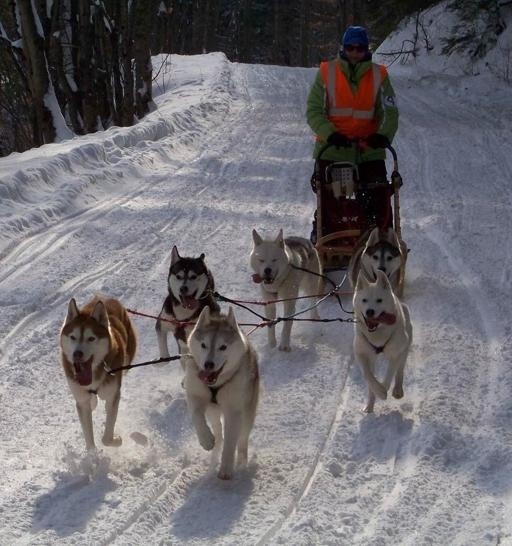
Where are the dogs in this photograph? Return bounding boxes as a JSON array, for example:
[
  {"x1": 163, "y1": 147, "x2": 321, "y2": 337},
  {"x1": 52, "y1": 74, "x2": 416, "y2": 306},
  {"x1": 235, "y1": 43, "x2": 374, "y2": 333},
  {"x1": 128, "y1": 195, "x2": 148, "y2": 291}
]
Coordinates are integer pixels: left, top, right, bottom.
[
  {"x1": 153, "y1": 245, "x2": 220, "y2": 371},
  {"x1": 181, "y1": 305, "x2": 261, "y2": 480},
  {"x1": 347, "y1": 227, "x2": 404, "y2": 299},
  {"x1": 249, "y1": 228, "x2": 321, "y2": 352},
  {"x1": 352, "y1": 268, "x2": 413, "y2": 413},
  {"x1": 59, "y1": 294, "x2": 137, "y2": 464}
]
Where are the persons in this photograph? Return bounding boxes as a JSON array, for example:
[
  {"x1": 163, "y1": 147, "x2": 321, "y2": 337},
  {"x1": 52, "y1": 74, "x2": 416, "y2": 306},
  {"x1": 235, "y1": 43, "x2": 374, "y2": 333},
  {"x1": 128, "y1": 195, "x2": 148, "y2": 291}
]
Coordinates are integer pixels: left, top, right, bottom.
[{"x1": 305, "y1": 25, "x2": 399, "y2": 244}]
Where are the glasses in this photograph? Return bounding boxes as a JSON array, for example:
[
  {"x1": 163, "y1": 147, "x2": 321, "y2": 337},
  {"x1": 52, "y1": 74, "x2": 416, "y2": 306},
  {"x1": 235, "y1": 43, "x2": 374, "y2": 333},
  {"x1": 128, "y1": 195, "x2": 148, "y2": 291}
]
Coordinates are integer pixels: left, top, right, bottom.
[{"x1": 344, "y1": 46, "x2": 366, "y2": 53}]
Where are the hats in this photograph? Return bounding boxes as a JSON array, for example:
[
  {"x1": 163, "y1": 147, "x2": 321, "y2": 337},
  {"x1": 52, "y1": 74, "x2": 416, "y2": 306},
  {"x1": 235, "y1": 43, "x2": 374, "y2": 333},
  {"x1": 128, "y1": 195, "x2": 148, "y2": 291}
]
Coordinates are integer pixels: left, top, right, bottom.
[{"x1": 342, "y1": 26, "x2": 369, "y2": 46}]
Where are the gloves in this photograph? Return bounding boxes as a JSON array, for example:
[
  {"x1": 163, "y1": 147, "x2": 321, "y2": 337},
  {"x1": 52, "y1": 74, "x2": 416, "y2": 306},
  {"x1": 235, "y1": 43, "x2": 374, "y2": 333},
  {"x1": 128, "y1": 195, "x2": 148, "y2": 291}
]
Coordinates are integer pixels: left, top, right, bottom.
[
  {"x1": 367, "y1": 133, "x2": 390, "y2": 148},
  {"x1": 327, "y1": 131, "x2": 352, "y2": 148}
]
